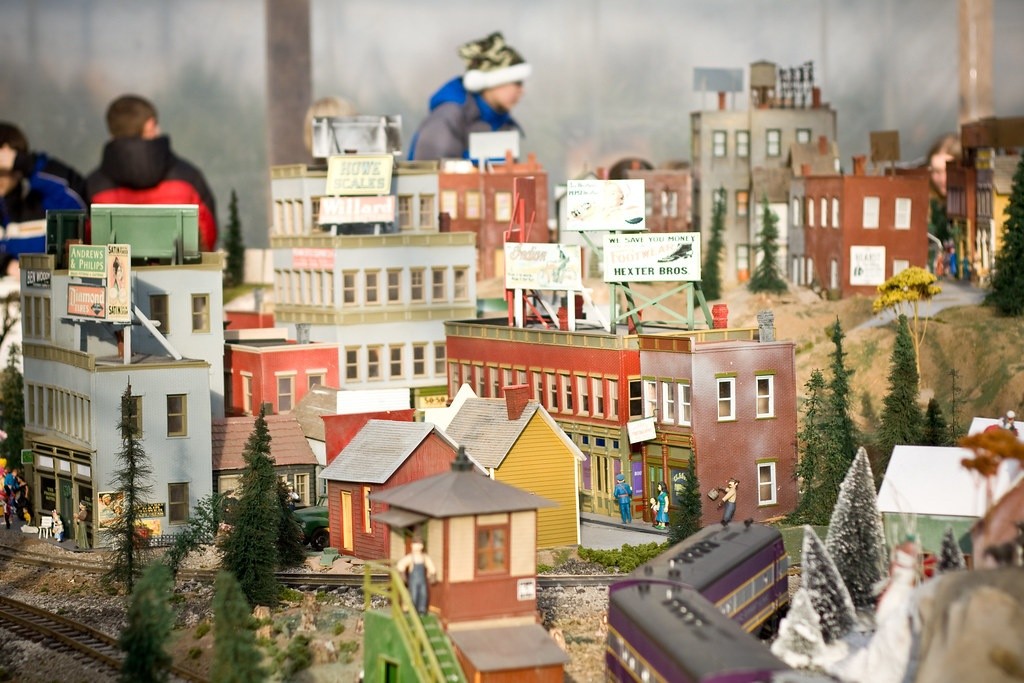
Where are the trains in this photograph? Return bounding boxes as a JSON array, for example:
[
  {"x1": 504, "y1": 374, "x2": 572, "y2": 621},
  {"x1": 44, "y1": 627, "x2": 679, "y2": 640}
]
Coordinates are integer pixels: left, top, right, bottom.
[{"x1": 604, "y1": 522, "x2": 795, "y2": 683}]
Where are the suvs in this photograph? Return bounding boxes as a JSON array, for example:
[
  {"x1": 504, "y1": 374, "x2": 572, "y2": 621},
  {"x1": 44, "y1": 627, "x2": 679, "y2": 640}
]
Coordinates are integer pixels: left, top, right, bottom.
[{"x1": 292, "y1": 494, "x2": 329, "y2": 551}]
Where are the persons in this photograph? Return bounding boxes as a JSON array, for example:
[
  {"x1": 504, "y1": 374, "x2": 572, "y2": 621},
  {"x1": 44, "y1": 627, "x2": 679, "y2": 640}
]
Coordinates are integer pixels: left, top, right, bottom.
[
  {"x1": 0, "y1": 93, "x2": 359, "y2": 284},
  {"x1": 717, "y1": 477, "x2": 740, "y2": 524},
  {"x1": 4, "y1": 469, "x2": 90, "y2": 549},
  {"x1": 927, "y1": 132, "x2": 960, "y2": 275},
  {"x1": 615, "y1": 475, "x2": 633, "y2": 524},
  {"x1": 408, "y1": 31, "x2": 530, "y2": 162},
  {"x1": 934, "y1": 246, "x2": 975, "y2": 283},
  {"x1": 395, "y1": 539, "x2": 437, "y2": 615},
  {"x1": 650, "y1": 481, "x2": 669, "y2": 530}
]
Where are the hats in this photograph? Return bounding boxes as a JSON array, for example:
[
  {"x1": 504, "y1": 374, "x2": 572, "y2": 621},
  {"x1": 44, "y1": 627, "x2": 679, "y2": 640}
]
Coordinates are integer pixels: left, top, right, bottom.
[{"x1": 456, "y1": 30, "x2": 534, "y2": 92}]
